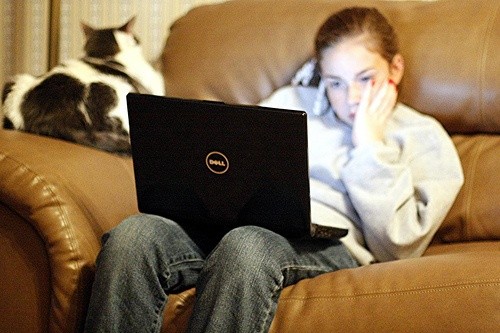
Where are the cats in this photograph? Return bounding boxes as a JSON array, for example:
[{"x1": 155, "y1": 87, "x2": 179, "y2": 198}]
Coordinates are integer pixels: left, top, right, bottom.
[{"x1": 2, "y1": 13, "x2": 166, "y2": 157}]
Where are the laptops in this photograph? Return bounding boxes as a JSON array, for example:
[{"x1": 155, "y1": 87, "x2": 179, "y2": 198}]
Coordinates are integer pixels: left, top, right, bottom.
[{"x1": 125, "y1": 92, "x2": 348, "y2": 247}]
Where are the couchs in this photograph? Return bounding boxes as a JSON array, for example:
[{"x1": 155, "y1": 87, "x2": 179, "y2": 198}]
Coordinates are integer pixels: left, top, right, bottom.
[{"x1": 0, "y1": 0, "x2": 499, "y2": 332}]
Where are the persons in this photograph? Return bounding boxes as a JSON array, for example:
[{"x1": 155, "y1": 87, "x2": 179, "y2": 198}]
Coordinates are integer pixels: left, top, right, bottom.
[{"x1": 83, "y1": 8, "x2": 464, "y2": 333}]
